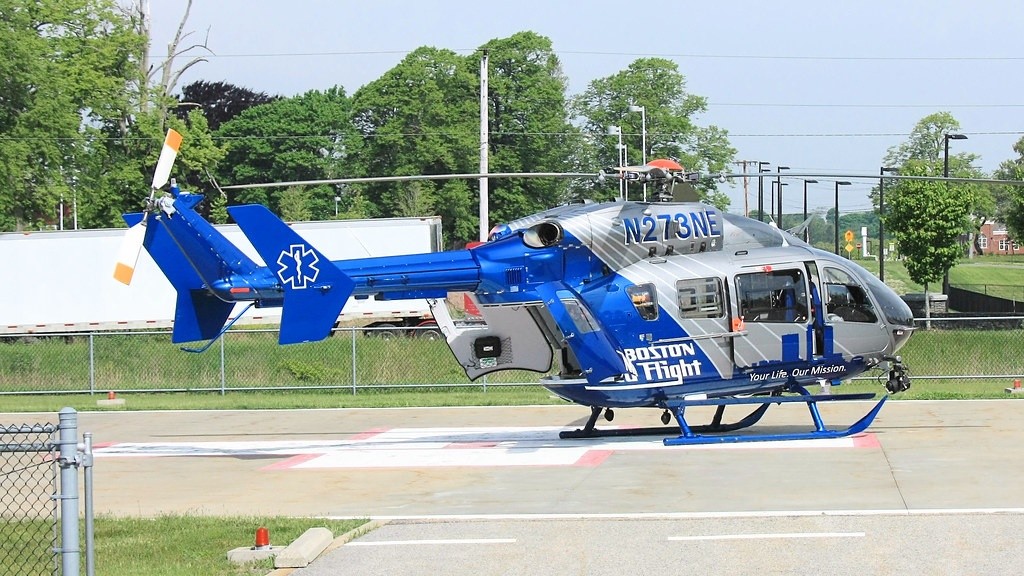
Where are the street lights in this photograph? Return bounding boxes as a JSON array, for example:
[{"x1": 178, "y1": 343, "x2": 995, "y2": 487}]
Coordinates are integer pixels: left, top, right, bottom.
[
  {"x1": 804, "y1": 180, "x2": 819, "y2": 244},
  {"x1": 879, "y1": 167, "x2": 899, "y2": 283},
  {"x1": 616, "y1": 145, "x2": 628, "y2": 203},
  {"x1": 608, "y1": 125, "x2": 629, "y2": 201},
  {"x1": 771, "y1": 166, "x2": 789, "y2": 231},
  {"x1": 835, "y1": 181, "x2": 853, "y2": 254},
  {"x1": 630, "y1": 106, "x2": 647, "y2": 201},
  {"x1": 943, "y1": 134, "x2": 969, "y2": 312},
  {"x1": 758, "y1": 162, "x2": 770, "y2": 221}
]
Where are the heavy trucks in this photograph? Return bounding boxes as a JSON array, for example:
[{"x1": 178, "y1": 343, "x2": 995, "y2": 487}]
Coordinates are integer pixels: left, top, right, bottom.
[{"x1": 0, "y1": 216, "x2": 499, "y2": 345}]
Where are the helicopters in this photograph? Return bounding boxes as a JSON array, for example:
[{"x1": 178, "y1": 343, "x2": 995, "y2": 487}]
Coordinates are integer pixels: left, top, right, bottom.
[{"x1": 112, "y1": 128, "x2": 916, "y2": 446}]
